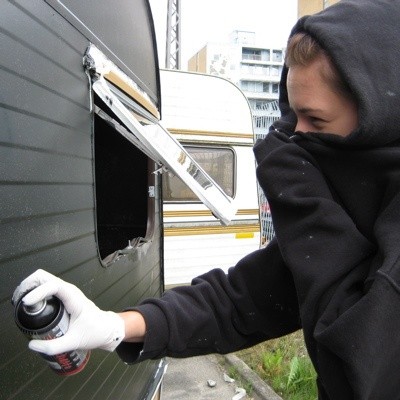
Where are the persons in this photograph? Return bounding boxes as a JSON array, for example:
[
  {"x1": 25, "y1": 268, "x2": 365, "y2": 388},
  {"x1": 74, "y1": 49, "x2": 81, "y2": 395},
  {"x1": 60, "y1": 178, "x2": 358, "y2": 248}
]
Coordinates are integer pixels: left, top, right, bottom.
[{"x1": 12, "y1": 0, "x2": 400, "y2": 400}]
